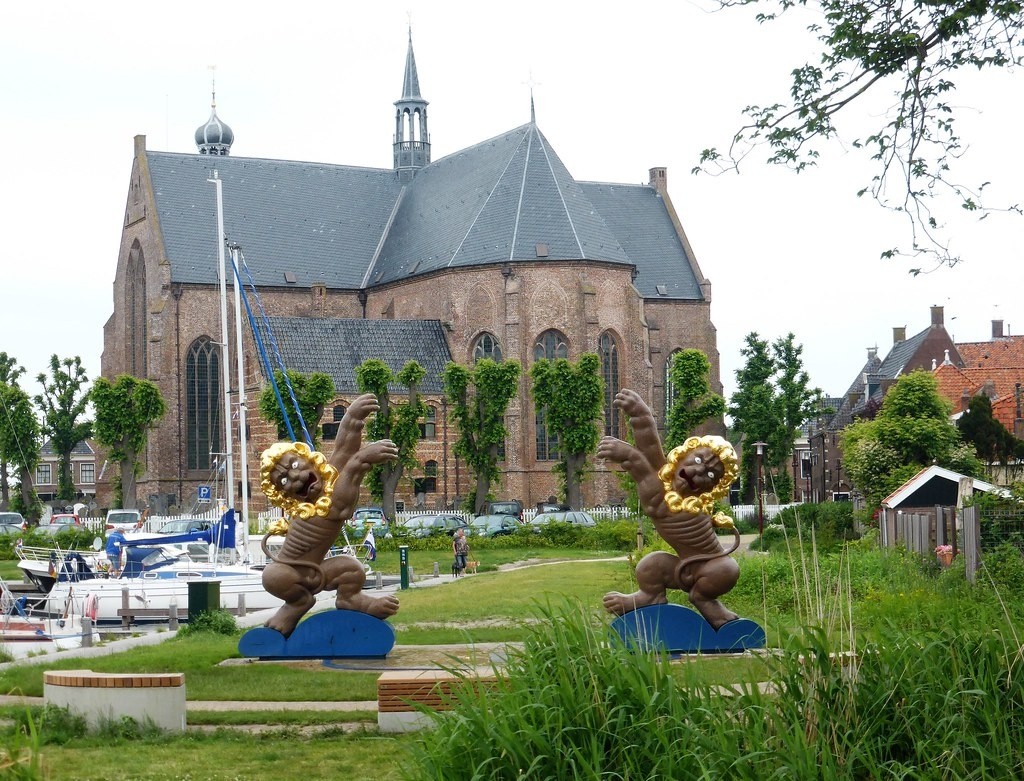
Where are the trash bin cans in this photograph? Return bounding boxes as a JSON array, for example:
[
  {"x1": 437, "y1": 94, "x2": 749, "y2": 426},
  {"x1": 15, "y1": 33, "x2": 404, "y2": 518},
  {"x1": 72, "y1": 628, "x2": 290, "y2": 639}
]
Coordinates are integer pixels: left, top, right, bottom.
[{"x1": 187, "y1": 581, "x2": 221, "y2": 623}]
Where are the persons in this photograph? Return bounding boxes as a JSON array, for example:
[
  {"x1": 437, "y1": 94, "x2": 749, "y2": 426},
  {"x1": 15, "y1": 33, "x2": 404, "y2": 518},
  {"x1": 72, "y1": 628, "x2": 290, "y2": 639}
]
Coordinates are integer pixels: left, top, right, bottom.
[
  {"x1": 452, "y1": 528, "x2": 470, "y2": 577},
  {"x1": 104, "y1": 527, "x2": 127, "y2": 579},
  {"x1": 93, "y1": 558, "x2": 101, "y2": 571}
]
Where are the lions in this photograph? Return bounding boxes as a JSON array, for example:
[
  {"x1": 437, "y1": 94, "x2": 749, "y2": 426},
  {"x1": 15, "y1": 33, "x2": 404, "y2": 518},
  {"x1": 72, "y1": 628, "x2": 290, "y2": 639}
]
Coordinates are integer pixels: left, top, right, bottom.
[
  {"x1": 596, "y1": 388, "x2": 741, "y2": 632},
  {"x1": 260, "y1": 393, "x2": 400, "y2": 640}
]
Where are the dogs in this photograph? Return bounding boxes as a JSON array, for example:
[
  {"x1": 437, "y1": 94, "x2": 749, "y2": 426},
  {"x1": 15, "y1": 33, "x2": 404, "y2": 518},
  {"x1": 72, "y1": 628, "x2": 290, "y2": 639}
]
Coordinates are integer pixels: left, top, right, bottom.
[{"x1": 452, "y1": 561, "x2": 481, "y2": 578}]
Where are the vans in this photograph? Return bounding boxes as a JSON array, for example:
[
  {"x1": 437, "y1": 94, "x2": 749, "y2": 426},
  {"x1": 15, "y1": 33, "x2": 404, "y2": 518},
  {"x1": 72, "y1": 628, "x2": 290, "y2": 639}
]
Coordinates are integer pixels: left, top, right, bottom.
[{"x1": 103, "y1": 509, "x2": 144, "y2": 534}]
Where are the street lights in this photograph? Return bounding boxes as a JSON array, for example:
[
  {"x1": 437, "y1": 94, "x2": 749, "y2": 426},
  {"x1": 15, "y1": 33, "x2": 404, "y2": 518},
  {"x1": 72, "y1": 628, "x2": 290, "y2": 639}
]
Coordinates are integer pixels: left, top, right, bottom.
[{"x1": 751, "y1": 439, "x2": 770, "y2": 535}]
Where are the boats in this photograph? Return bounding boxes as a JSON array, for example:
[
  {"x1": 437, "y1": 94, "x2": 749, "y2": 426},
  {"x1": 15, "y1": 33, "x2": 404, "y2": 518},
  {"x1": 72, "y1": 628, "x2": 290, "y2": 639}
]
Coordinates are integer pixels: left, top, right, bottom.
[
  {"x1": 0, "y1": 578, "x2": 99, "y2": 660},
  {"x1": 44, "y1": 572, "x2": 341, "y2": 623},
  {"x1": 17, "y1": 532, "x2": 216, "y2": 592}
]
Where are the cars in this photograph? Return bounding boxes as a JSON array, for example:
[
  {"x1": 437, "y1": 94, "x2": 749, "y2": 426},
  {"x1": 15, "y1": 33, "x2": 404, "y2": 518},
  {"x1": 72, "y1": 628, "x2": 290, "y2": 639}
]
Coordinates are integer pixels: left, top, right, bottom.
[
  {"x1": 397, "y1": 514, "x2": 469, "y2": 539},
  {"x1": 350, "y1": 507, "x2": 388, "y2": 537},
  {"x1": 156, "y1": 520, "x2": 213, "y2": 534},
  {"x1": 33, "y1": 525, "x2": 92, "y2": 542},
  {"x1": 455, "y1": 516, "x2": 526, "y2": 539},
  {"x1": 529, "y1": 511, "x2": 596, "y2": 529},
  {"x1": 49, "y1": 514, "x2": 79, "y2": 524},
  {"x1": 0, "y1": 512, "x2": 26, "y2": 539}
]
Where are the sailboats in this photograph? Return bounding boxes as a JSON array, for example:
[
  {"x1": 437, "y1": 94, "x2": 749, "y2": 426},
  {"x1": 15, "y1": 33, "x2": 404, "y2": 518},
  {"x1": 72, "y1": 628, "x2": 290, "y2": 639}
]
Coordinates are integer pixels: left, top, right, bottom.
[{"x1": 122, "y1": 169, "x2": 363, "y2": 613}]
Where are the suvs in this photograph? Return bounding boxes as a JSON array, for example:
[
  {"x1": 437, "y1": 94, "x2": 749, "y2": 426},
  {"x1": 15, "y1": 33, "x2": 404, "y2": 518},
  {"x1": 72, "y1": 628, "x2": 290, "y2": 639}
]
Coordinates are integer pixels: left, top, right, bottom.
[{"x1": 478, "y1": 498, "x2": 523, "y2": 521}]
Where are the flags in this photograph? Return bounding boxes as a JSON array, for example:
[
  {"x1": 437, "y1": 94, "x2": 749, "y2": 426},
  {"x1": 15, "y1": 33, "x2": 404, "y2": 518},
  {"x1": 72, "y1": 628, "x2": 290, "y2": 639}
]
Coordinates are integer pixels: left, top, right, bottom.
[
  {"x1": 48, "y1": 557, "x2": 57, "y2": 578},
  {"x1": 363, "y1": 528, "x2": 376, "y2": 562}
]
